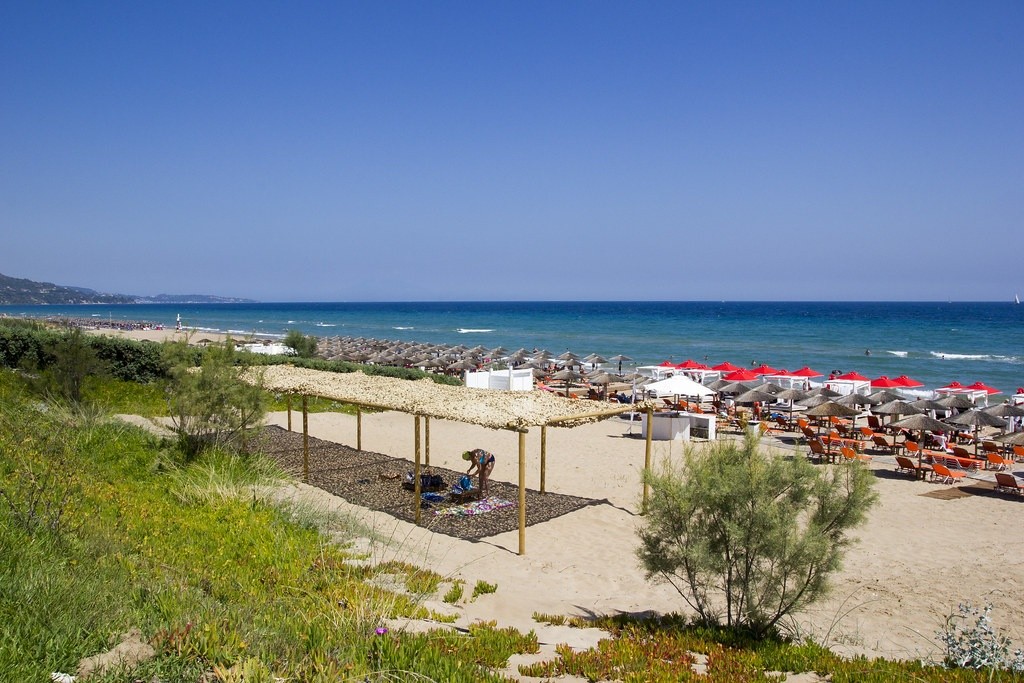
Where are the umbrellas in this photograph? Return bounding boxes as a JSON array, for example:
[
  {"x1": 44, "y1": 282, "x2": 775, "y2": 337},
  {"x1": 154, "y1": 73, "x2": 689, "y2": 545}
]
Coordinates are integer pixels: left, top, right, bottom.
[{"x1": 307, "y1": 333, "x2": 1024, "y2": 480}]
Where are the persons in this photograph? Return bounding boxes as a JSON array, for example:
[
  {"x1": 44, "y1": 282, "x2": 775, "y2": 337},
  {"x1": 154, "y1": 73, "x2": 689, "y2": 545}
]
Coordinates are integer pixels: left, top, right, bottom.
[
  {"x1": 1014, "y1": 420, "x2": 1022, "y2": 433},
  {"x1": 610, "y1": 389, "x2": 621, "y2": 402},
  {"x1": 803, "y1": 383, "x2": 806, "y2": 390},
  {"x1": 864, "y1": 348, "x2": 871, "y2": 356},
  {"x1": 924, "y1": 434, "x2": 935, "y2": 445},
  {"x1": 808, "y1": 382, "x2": 812, "y2": 390},
  {"x1": 553, "y1": 363, "x2": 558, "y2": 369},
  {"x1": 704, "y1": 355, "x2": 708, "y2": 360},
  {"x1": 967, "y1": 429, "x2": 974, "y2": 444},
  {"x1": 751, "y1": 360, "x2": 757, "y2": 366},
  {"x1": 760, "y1": 410, "x2": 766, "y2": 416},
  {"x1": 462, "y1": 449, "x2": 496, "y2": 499},
  {"x1": 910, "y1": 429, "x2": 920, "y2": 440}
]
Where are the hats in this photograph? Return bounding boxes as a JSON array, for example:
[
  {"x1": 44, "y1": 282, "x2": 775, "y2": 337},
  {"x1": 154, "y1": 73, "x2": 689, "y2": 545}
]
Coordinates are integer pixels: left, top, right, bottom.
[{"x1": 462, "y1": 451, "x2": 470, "y2": 460}]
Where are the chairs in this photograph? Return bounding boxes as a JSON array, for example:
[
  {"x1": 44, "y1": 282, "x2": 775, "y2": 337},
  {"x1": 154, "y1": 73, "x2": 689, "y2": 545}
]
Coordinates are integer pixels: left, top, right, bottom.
[{"x1": 537, "y1": 364, "x2": 1024, "y2": 496}]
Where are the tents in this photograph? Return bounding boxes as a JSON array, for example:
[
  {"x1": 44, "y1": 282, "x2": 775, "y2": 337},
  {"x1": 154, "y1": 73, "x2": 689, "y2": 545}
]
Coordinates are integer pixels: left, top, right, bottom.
[
  {"x1": 762, "y1": 374, "x2": 808, "y2": 391},
  {"x1": 933, "y1": 387, "x2": 988, "y2": 420},
  {"x1": 822, "y1": 378, "x2": 872, "y2": 410},
  {"x1": 678, "y1": 368, "x2": 722, "y2": 386},
  {"x1": 635, "y1": 366, "x2": 675, "y2": 382}
]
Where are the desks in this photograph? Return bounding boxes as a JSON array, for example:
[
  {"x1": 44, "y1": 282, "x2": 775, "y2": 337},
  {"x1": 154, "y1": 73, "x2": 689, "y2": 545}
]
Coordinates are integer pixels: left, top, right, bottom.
[
  {"x1": 1004, "y1": 451, "x2": 1014, "y2": 460},
  {"x1": 977, "y1": 457, "x2": 989, "y2": 470},
  {"x1": 851, "y1": 431, "x2": 861, "y2": 439},
  {"x1": 450, "y1": 489, "x2": 481, "y2": 503},
  {"x1": 894, "y1": 445, "x2": 906, "y2": 457},
  {"x1": 401, "y1": 483, "x2": 449, "y2": 492},
  {"x1": 916, "y1": 468, "x2": 934, "y2": 481},
  {"x1": 639, "y1": 412, "x2": 717, "y2": 442}
]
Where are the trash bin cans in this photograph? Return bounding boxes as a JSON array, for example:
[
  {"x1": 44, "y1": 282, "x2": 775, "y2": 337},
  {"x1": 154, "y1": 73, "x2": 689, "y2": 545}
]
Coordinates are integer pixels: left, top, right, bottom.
[
  {"x1": 746, "y1": 420, "x2": 760, "y2": 439},
  {"x1": 725, "y1": 396, "x2": 734, "y2": 408}
]
